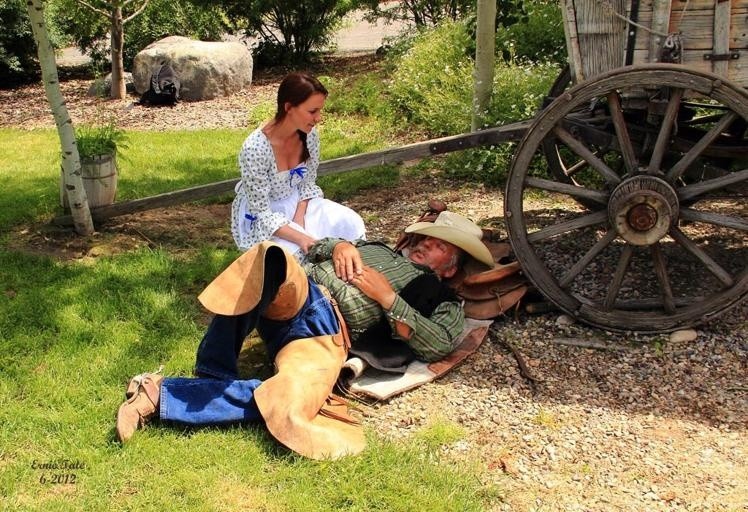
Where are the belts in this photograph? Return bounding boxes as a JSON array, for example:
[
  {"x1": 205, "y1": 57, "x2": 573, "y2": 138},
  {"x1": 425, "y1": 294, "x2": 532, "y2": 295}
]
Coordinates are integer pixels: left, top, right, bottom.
[{"x1": 317, "y1": 284, "x2": 352, "y2": 352}]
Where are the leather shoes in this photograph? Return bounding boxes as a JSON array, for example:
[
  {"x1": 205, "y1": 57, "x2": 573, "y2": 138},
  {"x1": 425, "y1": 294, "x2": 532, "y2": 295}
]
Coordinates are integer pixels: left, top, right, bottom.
[{"x1": 116, "y1": 372, "x2": 163, "y2": 443}]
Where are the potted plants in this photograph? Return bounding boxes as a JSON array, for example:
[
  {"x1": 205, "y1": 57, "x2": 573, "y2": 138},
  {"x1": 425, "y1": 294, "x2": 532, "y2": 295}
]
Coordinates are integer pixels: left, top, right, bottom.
[{"x1": 56, "y1": 59, "x2": 135, "y2": 215}]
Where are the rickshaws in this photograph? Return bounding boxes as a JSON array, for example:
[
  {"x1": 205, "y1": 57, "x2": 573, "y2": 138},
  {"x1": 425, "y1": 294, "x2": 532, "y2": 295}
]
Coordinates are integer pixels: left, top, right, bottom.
[{"x1": 50, "y1": 0, "x2": 747, "y2": 335}]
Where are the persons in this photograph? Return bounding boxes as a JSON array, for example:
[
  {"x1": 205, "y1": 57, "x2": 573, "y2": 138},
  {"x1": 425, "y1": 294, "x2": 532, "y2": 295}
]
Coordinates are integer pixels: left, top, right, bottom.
[
  {"x1": 230, "y1": 70, "x2": 367, "y2": 256},
  {"x1": 115, "y1": 210, "x2": 495, "y2": 446}
]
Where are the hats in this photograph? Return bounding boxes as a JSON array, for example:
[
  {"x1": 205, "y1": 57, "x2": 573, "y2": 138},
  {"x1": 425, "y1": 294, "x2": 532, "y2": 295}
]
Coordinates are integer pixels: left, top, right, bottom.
[{"x1": 404, "y1": 210, "x2": 495, "y2": 268}]
